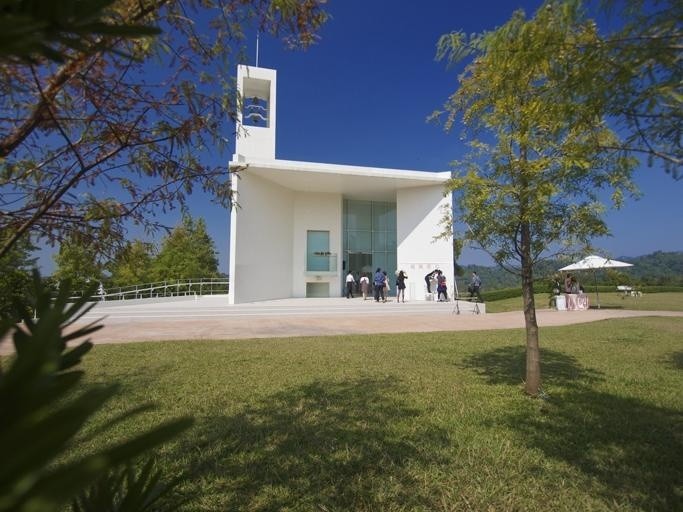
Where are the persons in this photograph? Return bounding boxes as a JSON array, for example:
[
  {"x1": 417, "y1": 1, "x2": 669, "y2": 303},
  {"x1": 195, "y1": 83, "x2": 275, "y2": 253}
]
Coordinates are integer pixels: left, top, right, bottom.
[
  {"x1": 437, "y1": 270, "x2": 448, "y2": 301},
  {"x1": 345, "y1": 270, "x2": 356, "y2": 297},
  {"x1": 397, "y1": 271, "x2": 408, "y2": 302},
  {"x1": 549, "y1": 274, "x2": 584, "y2": 309},
  {"x1": 373, "y1": 268, "x2": 391, "y2": 302},
  {"x1": 425, "y1": 269, "x2": 438, "y2": 293},
  {"x1": 359, "y1": 272, "x2": 370, "y2": 299},
  {"x1": 467, "y1": 272, "x2": 483, "y2": 303}
]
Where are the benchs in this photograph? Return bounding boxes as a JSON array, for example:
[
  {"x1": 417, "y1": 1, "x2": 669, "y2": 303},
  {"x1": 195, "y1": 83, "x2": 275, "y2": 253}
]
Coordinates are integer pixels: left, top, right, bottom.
[{"x1": 616, "y1": 285, "x2": 632, "y2": 295}]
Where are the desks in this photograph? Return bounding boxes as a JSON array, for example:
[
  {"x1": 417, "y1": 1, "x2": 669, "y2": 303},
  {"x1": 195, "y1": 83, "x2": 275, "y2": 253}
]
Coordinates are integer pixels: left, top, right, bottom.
[{"x1": 548, "y1": 293, "x2": 589, "y2": 311}]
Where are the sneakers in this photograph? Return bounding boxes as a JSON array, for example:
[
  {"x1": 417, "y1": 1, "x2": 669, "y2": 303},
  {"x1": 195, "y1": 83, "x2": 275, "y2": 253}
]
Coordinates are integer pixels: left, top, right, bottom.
[
  {"x1": 437, "y1": 299, "x2": 448, "y2": 302},
  {"x1": 373, "y1": 297, "x2": 388, "y2": 303},
  {"x1": 347, "y1": 296, "x2": 354, "y2": 298},
  {"x1": 397, "y1": 299, "x2": 405, "y2": 303}
]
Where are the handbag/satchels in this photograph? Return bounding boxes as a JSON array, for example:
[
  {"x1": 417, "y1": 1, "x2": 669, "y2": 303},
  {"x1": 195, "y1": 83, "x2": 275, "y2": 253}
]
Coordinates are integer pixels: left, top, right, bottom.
[
  {"x1": 396, "y1": 275, "x2": 404, "y2": 286},
  {"x1": 468, "y1": 285, "x2": 472, "y2": 293},
  {"x1": 375, "y1": 281, "x2": 386, "y2": 287}
]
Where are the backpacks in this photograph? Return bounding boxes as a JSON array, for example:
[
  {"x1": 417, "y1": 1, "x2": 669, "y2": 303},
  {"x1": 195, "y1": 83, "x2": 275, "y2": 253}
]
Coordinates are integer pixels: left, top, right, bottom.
[{"x1": 440, "y1": 278, "x2": 446, "y2": 288}]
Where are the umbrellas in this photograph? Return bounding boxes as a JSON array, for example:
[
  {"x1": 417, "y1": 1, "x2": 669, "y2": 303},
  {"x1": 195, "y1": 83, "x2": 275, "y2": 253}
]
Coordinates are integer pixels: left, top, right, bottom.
[{"x1": 558, "y1": 255, "x2": 635, "y2": 308}]
